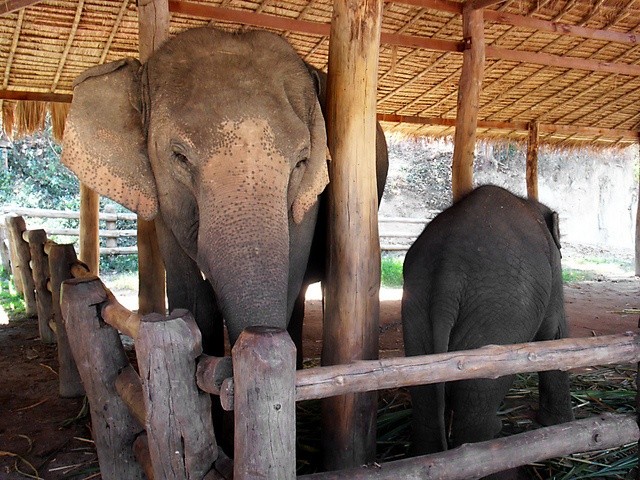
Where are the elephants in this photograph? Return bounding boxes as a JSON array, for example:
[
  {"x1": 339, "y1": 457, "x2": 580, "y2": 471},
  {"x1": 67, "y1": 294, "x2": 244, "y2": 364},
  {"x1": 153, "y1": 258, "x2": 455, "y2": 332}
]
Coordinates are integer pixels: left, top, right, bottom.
[
  {"x1": 60, "y1": 25, "x2": 389, "y2": 442},
  {"x1": 401, "y1": 184, "x2": 576, "y2": 479}
]
